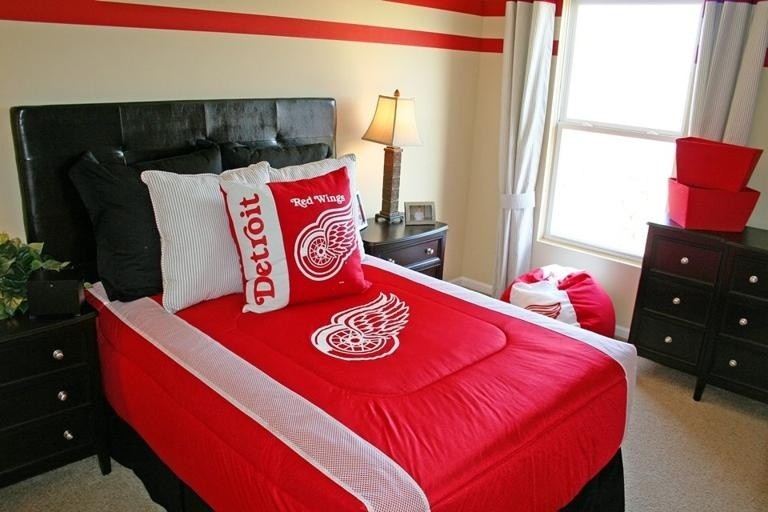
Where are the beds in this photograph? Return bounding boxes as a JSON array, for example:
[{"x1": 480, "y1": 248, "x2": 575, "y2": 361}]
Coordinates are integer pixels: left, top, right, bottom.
[{"x1": 7, "y1": 99, "x2": 630, "y2": 512}]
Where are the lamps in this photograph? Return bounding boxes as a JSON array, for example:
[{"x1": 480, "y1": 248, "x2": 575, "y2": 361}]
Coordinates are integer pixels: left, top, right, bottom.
[{"x1": 360, "y1": 86, "x2": 425, "y2": 225}]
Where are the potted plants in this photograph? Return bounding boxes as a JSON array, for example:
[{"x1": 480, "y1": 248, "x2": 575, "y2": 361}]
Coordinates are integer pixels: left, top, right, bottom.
[{"x1": 0, "y1": 229, "x2": 66, "y2": 328}]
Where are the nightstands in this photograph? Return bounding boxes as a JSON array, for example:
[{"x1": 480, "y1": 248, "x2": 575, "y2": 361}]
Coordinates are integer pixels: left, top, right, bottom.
[
  {"x1": 1, "y1": 303, "x2": 111, "y2": 488},
  {"x1": 357, "y1": 216, "x2": 450, "y2": 283}
]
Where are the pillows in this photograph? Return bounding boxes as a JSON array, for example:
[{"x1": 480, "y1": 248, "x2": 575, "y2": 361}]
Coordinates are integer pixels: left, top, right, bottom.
[{"x1": 69, "y1": 138, "x2": 372, "y2": 315}]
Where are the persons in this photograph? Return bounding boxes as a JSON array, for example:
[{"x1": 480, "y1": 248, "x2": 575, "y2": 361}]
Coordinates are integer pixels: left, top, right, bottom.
[{"x1": 412, "y1": 206, "x2": 425, "y2": 222}]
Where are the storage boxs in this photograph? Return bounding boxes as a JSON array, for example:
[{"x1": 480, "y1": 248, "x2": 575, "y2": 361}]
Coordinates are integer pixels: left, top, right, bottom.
[
  {"x1": 665, "y1": 176, "x2": 760, "y2": 234},
  {"x1": 676, "y1": 134, "x2": 763, "y2": 192}
]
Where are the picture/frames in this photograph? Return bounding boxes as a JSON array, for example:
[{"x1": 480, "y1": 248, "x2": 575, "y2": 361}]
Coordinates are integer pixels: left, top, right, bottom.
[{"x1": 404, "y1": 200, "x2": 437, "y2": 225}]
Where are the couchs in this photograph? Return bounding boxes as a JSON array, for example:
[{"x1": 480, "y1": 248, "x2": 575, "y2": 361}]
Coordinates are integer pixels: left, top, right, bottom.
[{"x1": 497, "y1": 261, "x2": 619, "y2": 341}]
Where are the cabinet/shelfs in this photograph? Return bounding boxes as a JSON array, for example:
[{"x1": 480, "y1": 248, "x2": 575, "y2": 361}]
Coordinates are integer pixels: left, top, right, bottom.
[{"x1": 625, "y1": 219, "x2": 768, "y2": 407}]
